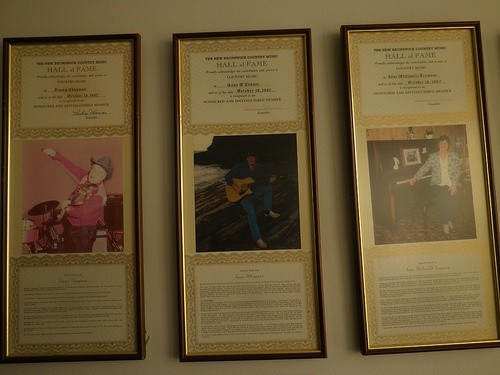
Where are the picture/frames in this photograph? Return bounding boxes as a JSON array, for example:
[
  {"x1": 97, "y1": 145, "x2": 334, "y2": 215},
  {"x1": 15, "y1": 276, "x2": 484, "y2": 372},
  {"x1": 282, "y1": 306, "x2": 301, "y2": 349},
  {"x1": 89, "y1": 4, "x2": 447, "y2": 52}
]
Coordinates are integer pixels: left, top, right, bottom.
[
  {"x1": 0, "y1": 33, "x2": 146, "y2": 364},
  {"x1": 340, "y1": 20, "x2": 500, "y2": 357},
  {"x1": 172, "y1": 27, "x2": 328, "y2": 362}
]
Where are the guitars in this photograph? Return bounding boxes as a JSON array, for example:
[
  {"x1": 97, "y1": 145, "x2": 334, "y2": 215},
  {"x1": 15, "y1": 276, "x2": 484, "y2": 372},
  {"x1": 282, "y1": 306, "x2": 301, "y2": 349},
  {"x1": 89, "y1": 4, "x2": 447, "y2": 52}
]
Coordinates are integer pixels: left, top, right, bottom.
[{"x1": 226, "y1": 175, "x2": 286, "y2": 203}]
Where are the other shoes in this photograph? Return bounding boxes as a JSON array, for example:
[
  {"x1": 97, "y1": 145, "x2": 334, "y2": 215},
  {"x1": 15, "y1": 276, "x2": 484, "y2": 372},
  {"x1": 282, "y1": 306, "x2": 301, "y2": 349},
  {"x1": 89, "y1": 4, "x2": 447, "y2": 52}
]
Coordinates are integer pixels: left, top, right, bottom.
[
  {"x1": 264, "y1": 211, "x2": 280, "y2": 218},
  {"x1": 450, "y1": 228, "x2": 455, "y2": 234},
  {"x1": 255, "y1": 238, "x2": 267, "y2": 249},
  {"x1": 442, "y1": 230, "x2": 450, "y2": 239}
]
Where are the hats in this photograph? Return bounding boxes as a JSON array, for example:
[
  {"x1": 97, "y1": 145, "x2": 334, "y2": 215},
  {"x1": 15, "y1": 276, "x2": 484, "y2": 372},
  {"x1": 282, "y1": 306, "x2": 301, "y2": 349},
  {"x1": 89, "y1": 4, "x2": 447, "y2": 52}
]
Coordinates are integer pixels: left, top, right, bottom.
[{"x1": 89, "y1": 157, "x2": 113, "y2": 179}]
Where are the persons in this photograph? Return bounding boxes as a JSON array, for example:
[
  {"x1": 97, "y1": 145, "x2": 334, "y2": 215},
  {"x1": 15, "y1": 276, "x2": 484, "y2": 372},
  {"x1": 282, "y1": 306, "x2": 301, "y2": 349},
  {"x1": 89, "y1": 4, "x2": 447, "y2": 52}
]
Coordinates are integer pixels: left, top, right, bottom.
[
  {"x1": 225, "y1": 147, "x2": 281, "y2": 250},
  {"x1": 43, "y1": 144, "x2": 114, "y2": 253},
  {"x1": 409, "y1": 135, "x2": 464, "y2": 240}
]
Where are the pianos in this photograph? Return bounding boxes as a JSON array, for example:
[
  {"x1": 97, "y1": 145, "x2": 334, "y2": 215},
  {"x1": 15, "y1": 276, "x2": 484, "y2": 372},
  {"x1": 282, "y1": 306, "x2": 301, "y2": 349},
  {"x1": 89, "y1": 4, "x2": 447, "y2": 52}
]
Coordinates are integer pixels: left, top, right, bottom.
[{"x1": 367, "y1": 139, "x2": 440, "y2": 229}]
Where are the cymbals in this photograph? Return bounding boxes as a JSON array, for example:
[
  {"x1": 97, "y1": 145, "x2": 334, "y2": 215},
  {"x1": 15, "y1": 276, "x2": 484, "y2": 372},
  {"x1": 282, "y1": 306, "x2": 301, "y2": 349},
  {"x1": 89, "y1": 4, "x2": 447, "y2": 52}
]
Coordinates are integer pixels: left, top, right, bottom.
[{"x1": 28, "y1": 200, "x2": 59, "y2": 216}]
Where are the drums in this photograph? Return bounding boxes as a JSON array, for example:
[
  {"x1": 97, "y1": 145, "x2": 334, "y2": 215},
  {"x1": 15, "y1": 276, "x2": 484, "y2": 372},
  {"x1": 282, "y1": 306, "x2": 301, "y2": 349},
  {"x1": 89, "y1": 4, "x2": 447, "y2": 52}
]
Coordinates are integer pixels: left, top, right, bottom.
[
  {"x1": 21, "y1": 218, "x2": 36, "y2": 229},
  {"x1": 22, "y1": 228, "x2": 39, "y2": 243}
]
